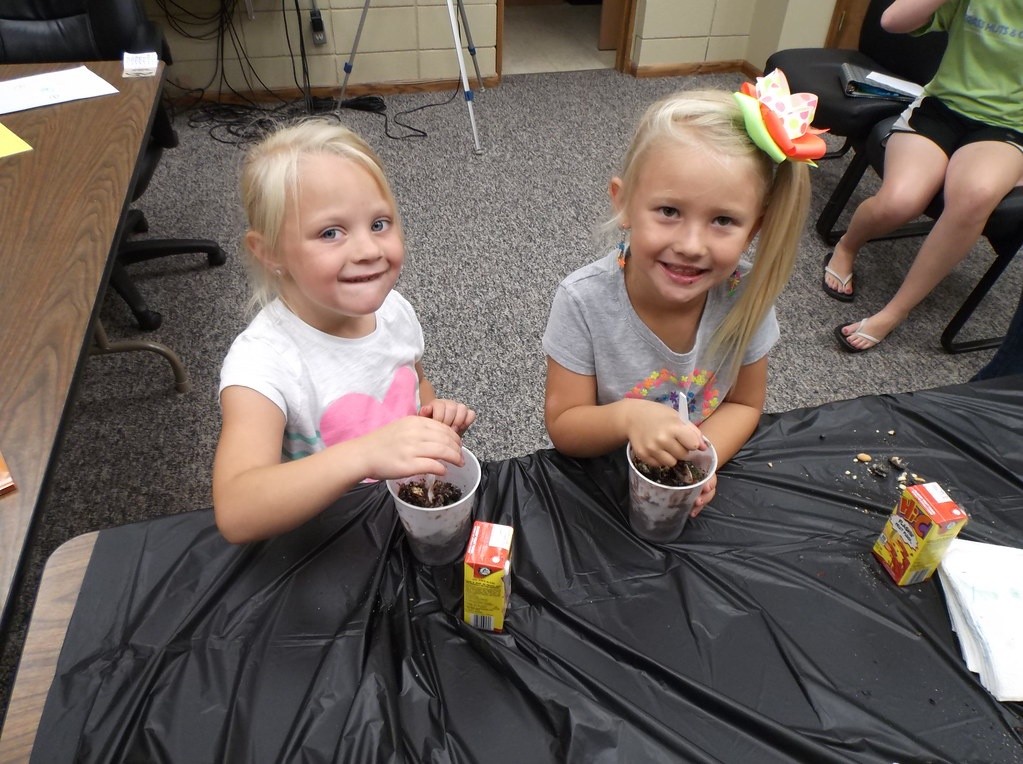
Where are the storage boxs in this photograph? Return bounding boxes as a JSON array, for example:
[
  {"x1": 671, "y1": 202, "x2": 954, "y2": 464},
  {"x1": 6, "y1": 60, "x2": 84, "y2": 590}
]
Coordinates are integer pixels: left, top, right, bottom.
[
  {"x1": 872, "y1": 481, "x2": 967, "y2": 586},
  {"x1": 464, "y1": 521, "x2": 513, "y2": 633}
]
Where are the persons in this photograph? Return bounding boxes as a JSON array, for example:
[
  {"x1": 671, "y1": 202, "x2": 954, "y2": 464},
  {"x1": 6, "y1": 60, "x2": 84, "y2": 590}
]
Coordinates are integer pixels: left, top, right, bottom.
[
  {"x1": 212, "y1": 121, "x2": 476, "y2": 544},
  {"x1": 542, "y1": 89, "x2": 825, "y2": 517},
  {"x1": 822, "y1": 0, "x2": 1023, "y2": 352}
]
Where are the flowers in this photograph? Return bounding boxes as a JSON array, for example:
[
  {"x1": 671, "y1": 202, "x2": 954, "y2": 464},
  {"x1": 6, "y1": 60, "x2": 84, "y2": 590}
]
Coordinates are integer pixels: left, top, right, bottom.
[{"x1": 733, "y1": 68, "x2": 831, "y2": 168}]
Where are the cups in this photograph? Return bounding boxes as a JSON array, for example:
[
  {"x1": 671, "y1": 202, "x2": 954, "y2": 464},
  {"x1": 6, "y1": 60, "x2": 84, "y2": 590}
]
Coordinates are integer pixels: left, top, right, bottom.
[
  {"x1": 626, "y1": 430, "x2": 718, "y2": 545},
  {"x1": 387, "y1": 446, "x2": 482, "y2": 565}
]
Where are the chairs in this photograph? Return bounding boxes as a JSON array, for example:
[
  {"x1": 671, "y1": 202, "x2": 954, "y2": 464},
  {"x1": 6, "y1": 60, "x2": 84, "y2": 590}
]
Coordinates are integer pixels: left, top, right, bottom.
[
  {"x1": 0, "y1": 1, "x2": 229, "y2": 334},
  {"x1": 764, "y1": 0, "x2": 1023, "y2": 356}
]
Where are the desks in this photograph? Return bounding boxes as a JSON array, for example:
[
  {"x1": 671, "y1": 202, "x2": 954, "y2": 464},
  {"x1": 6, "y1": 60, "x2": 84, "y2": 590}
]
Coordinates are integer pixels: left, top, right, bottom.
[
  {"x1": 0, "y1": 373, "x2": 1023, "y2": 764},
  {"x1": 0, "y1": 58, "x2": 168, "y2": 620}
]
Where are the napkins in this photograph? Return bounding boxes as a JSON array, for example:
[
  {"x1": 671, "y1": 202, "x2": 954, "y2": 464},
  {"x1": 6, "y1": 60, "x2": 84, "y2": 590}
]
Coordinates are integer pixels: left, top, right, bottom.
[{"x1": 936, "y1": 537, "x2": 1023, "y2": 703}]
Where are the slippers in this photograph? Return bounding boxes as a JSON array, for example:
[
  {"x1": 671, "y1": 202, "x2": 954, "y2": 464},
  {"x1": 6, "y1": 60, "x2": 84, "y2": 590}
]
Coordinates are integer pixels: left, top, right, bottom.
[
  {"x1": 834, "y1": 317, "x2": 891, "y2": 351},
  {"x1": 821, "y1": 252, "x2": 854, "y2": 303}
]
